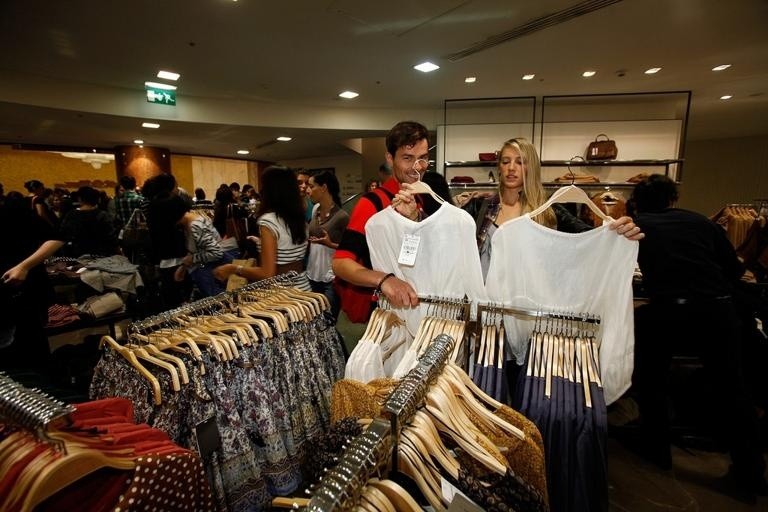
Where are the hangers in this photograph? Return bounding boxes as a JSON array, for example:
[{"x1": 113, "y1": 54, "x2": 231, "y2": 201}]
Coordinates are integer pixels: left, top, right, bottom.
[
  {"x1": 0, "y1": 270, "x2": 606, "y2": 512},
  {"x1": 392, "y1": 158, "x2": 445, "y2": 209},
  {"x1": 531, "y1": 157, "x2": 610, "y2": 223},
  {"x1": 708, "y1": 202, "x2": 768, "y2": 219}
]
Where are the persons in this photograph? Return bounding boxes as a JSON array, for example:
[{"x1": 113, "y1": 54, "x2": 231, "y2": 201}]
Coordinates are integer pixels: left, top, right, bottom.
[
  {"x1": 624, "y1": 172, "x2": 743, "y2": 307},
  {"x1": 2, "y1": 181, "x2": 68, "y2": 397},
  {"x1": 329, "y1": 121, "x2": 475, "y2": 308},
  {"x1": 459, "y1": 137, "x2": 646, "y2": 285},
  {"x1": 0, "y1": 161, "x2": 454, "y2": 313}
]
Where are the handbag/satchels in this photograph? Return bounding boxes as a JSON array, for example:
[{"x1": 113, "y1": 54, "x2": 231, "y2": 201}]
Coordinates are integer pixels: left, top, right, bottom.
[
  {"x1": 119, "y1": 208, "x2": 155, "y2": 255},
  {"x1": 587, "y1": 133, "x2": 618, "y2": 160},
  {"x1": 189, "y1": 250, "x2": 247, "y2": 297}
]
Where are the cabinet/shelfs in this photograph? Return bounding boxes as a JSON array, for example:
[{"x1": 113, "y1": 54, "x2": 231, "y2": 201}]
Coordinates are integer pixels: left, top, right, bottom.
[
  {"x1": 540, "y1": 90, "x2": 692, "y2": 190},
  {"x1": 443, "y1": 96, "x2": 535, "y2": 191}
]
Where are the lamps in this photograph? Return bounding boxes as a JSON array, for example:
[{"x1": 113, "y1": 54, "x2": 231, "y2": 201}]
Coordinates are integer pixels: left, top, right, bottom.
[{"x1": 50, "y1": 151, "x2": 115, "y2": 169}]
